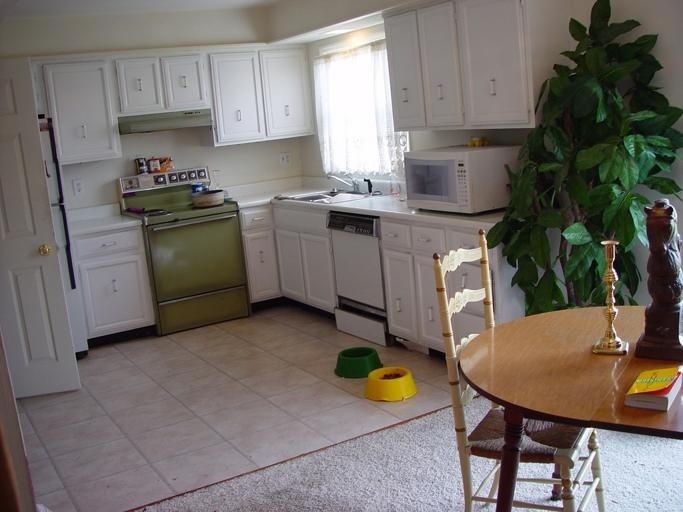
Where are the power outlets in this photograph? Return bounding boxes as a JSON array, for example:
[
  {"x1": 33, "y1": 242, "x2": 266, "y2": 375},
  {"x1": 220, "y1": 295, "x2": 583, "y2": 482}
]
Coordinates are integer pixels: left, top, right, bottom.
[
  {"x1": 72, "y1": 180, "x2": 85, "y2": 198},
  {"x1": 279, "y1": 152, "x2": 292, "y2": 168}
]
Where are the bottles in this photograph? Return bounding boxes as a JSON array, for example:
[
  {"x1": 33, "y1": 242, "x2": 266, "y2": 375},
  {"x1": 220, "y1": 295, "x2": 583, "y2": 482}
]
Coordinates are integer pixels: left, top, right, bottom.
[{"x1": 135, "y1": 156, "x2": 175, "y2": 174}]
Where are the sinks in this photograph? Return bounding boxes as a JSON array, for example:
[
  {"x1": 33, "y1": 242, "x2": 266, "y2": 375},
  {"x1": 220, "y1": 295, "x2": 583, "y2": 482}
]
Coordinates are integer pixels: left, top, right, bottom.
[{"x1": 291, "y1": 192, "x2": 328, "y2": 204}]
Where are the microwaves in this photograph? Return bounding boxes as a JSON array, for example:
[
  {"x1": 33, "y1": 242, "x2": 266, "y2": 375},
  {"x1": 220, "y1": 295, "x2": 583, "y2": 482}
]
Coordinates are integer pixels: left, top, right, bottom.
[{"x1": 401, "y1": 144, "x2": 523, "y2": 217}]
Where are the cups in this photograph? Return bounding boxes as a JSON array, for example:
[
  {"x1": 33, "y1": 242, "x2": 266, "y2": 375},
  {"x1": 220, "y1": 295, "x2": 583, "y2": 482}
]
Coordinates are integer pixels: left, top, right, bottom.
[{"x1": 465, "y1": 135, "x2": 491, "y2": 147}]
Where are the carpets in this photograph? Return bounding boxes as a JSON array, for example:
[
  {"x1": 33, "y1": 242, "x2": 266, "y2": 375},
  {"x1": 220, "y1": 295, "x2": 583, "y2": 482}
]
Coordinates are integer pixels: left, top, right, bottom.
[{"x1": 125, "y1": 393, "x2": 683, "y2": 512}]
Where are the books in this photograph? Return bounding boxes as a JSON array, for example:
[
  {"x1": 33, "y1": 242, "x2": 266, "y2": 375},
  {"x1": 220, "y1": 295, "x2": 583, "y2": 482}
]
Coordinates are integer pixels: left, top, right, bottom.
[{"x1": 624, "y1": 368, "x2": 682, "y2": 411}]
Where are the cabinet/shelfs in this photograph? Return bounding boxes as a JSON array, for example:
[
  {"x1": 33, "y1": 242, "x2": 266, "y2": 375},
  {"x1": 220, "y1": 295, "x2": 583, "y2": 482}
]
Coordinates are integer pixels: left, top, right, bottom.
[
  {"x1": 379, "y1": 217, "x2": 418, "y2": 344},
  {"x1": 416, "y1": 0, "x2": 464, "y2": 130},
  {"x1": 455, "y1": 0, "x2": 557, "y2": 129},
  {"x1": 384, "y1": 0, "x2": 427, "y2": 130},
  {"x1": 411, "y1": 214, "x2": 446, "y2": 353},
  {"x1": 115, "y1": 46, "x2": 210, "y2": 117},
  {"x1": 42, "y1": 50, "x2": 122, "y2": 166},
  {"x1": 270, "y1": 199, "x2": 335, "y2": 314},
  {"x1": 70, "y1": 221, "x2": 156, "y2": 340},
  {"x1": 446, "y1": 218, "x2": 545, "y2": 352},
  {"x1": 209, "y1": 43, "x2": 314, "y2": 148},
  {"x1": 238, "y1": 199, "x2": 281, "y2": 304}
]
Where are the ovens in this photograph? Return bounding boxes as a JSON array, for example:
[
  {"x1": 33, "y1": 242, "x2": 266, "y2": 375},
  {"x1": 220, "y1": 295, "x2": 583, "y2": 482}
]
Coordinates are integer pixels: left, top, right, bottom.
[{"x1": 143, "y1": 212, "x2": 250, "y2": 334}]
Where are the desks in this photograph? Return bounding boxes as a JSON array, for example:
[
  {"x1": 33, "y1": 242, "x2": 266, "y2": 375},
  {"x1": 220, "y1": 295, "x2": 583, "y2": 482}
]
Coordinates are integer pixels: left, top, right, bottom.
[{"x1": 459, "y1": 305, "x2": 683, "y2": 512}]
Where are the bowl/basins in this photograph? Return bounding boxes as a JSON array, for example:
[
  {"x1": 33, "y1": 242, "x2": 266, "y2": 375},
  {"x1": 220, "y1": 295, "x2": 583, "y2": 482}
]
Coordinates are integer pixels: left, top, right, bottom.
[
  {"x1": 361, "y1": 365, "x2": 419, "y2": 403},
  {"x1": 332, "y1": 345, "x2": 383, "y2": 377}
]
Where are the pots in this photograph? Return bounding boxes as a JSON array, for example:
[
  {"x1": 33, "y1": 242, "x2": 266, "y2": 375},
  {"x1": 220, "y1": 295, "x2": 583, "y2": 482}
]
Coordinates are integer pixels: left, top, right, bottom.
[{"x1": 191, "y1": 190, "x2": 225, "y2": 207}]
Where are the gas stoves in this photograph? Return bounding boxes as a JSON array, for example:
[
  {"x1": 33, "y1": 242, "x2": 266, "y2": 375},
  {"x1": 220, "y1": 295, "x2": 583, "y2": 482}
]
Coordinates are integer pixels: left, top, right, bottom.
[{"x1": 122, "y1": 188, "x2": 238, "y2": 225}]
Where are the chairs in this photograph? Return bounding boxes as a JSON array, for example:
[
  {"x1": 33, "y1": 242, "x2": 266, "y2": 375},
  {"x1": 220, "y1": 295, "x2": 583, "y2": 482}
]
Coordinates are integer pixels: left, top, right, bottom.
[{"x1": 433, "y1": 229, "x2": 606, "y2": 512}]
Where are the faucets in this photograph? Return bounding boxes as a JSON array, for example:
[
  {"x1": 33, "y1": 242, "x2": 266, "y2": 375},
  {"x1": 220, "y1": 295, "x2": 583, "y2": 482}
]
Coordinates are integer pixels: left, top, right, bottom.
[{"x1": 327, "y1": 174, "x2": 360, "y2": 191}]
